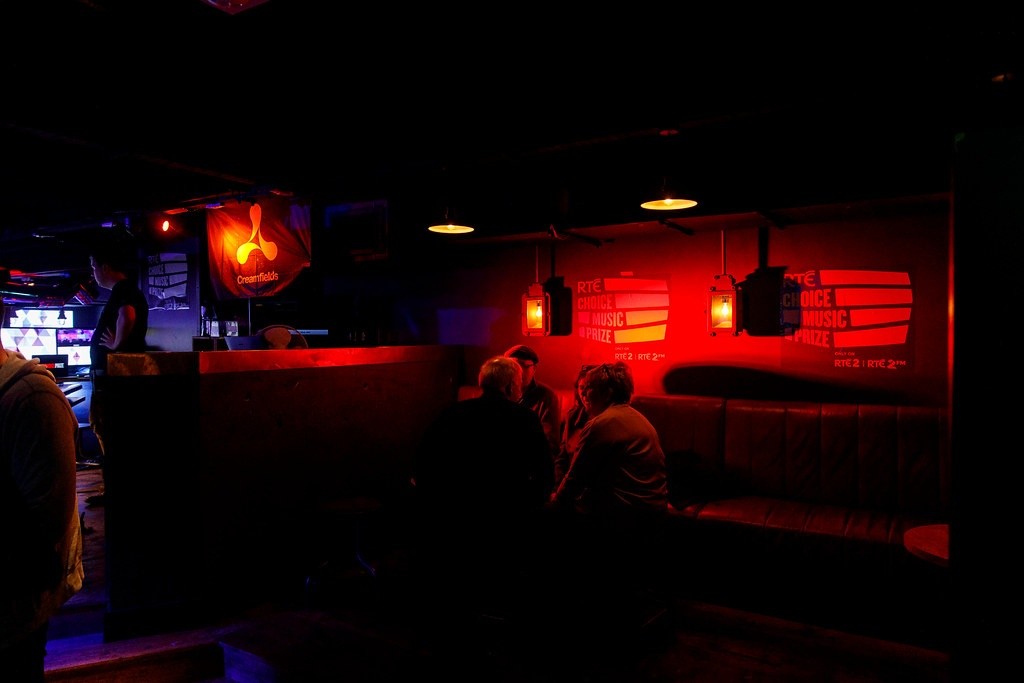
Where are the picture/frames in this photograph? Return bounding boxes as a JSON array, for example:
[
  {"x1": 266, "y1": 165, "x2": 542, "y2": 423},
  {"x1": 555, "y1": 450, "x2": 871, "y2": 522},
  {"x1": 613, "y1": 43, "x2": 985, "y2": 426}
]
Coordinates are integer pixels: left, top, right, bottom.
[{"x1": 321, "y1": 198, "x2": 390, "y2": 262}]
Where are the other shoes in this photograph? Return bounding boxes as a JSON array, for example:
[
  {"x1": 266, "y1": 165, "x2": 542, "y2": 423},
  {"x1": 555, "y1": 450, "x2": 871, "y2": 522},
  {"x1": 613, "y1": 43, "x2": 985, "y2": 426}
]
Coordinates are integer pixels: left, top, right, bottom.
[{"x1": 84, "y1": 493, "x2": 104, "y2": 505}]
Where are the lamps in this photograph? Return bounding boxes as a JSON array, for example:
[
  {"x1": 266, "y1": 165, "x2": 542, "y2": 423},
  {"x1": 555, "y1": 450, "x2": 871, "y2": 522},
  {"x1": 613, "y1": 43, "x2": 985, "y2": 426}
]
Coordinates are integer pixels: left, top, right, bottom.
[
  {"x1": 427, "y1": 198, "x2": 475, "y2": 234},
  {"x1": 704, "y1": 229, "x2": 744, "y2": 337},
  {"x1": 639, "y1": 133, "x2": 699, "y2": 209},
  {"x1": 521, "y1": 241, "x2": 553, "y2": 337}
]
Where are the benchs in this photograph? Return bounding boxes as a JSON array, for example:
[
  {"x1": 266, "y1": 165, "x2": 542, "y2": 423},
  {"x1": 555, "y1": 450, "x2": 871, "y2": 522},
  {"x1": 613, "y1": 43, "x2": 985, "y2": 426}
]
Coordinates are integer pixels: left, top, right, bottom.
[{"x1": 631, "y1": 393, "x2": 946, "y2": 545}]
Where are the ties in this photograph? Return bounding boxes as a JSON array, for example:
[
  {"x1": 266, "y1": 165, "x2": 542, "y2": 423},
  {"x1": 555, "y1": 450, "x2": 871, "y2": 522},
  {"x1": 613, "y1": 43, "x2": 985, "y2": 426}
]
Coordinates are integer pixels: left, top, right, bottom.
[{"x1": 523, "y1": 391, "x2": 528, "y2": 412}]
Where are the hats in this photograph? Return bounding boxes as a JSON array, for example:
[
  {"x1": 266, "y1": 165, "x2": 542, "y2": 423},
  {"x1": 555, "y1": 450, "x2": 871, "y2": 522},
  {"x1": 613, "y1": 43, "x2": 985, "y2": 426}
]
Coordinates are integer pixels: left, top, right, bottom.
[{"x1": 503, "y1": 345, "x2": 539, "y2": 365}]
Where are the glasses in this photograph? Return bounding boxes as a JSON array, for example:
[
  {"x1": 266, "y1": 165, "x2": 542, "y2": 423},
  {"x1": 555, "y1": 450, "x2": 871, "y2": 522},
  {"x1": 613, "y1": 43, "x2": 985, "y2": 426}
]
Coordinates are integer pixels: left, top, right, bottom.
[{"x1": 580, "y1": 364, "x2": 595, "y2": 371}]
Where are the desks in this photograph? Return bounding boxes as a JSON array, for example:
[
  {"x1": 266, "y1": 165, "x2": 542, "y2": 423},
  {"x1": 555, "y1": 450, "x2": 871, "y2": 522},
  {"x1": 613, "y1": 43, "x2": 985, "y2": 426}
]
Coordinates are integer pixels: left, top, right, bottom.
[{"x1": 903, "y1": 522, "x2": 950, "y2": 569}]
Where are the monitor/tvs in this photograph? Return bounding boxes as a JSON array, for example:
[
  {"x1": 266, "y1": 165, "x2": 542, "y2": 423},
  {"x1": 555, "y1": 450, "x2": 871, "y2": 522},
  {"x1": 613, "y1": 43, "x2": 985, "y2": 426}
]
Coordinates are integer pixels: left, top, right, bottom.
[{"x1": 0, "y1": 306, "x2": 95, "y2": 368}]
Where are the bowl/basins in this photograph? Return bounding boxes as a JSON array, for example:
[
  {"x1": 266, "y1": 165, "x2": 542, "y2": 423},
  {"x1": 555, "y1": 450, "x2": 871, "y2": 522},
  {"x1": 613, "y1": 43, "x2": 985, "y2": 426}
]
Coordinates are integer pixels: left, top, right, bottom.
[{"x1": 224, "y1": 336, "x2": 262, "y2": 350}]
